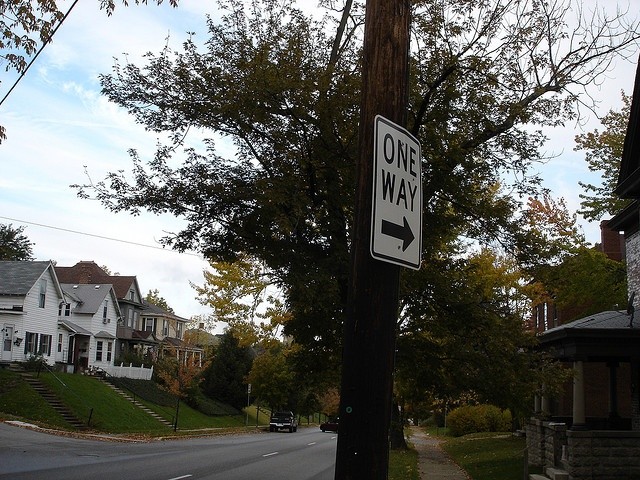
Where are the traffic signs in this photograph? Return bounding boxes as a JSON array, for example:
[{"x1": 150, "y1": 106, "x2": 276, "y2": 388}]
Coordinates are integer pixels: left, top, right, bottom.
[{"x1": 370, "y1": 113, "x2": 422, "y2": 271}]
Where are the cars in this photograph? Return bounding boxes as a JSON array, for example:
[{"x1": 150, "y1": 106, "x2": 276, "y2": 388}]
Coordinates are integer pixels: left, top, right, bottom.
[{"x1": 320, "y1": 421, "x2": 338, "y2": 433}]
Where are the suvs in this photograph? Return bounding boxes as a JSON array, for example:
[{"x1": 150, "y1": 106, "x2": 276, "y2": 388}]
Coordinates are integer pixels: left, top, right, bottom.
[{"x1": 269, "y1": 410, "x2": 298, "y2": 433}]
